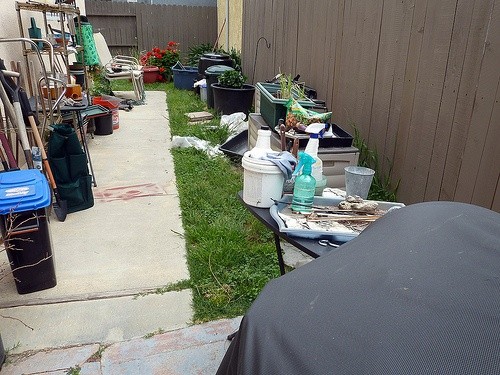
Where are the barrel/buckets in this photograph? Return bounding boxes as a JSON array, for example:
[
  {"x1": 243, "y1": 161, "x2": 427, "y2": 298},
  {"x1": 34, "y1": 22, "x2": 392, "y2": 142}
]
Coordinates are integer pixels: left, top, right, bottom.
[
  {"x1": 344, "y1": 166, "x2": 375, "y2": 200},
  {"x1": 90, "y1": 96, "x2": 119, "y2": 135},
  {"x1": 171, "y1": 66, "x2": 198, "y2": 90},
  {"x1": 241, "y1": 151, "x2": 284, "y2": 208}
]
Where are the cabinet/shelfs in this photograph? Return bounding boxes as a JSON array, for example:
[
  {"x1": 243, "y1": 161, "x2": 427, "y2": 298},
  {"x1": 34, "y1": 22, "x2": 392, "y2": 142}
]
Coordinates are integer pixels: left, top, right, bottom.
[{"x1": 15, "y1": 1, "x2": 95, "y2": 139}]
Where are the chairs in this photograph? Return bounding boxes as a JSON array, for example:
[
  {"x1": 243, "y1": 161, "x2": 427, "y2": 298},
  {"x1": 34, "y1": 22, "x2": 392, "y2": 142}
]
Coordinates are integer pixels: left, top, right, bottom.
[{"x1": 93, "y1": 32, "x2": 145, "y2": 101}]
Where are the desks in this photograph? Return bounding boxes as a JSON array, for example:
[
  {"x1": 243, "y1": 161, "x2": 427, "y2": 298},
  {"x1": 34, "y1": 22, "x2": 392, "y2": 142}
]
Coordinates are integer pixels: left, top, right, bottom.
[
  {"x1": 27, "y1": 93, "x2": 98, "y2": 188},
  {"x1": 237, "y1": 186, "x2": 357, "y2": 277}
]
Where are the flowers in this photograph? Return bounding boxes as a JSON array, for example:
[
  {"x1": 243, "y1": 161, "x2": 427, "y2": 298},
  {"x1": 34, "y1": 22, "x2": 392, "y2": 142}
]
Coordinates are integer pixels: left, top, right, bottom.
[{"x1": 139, "y1": 42, "x2": 182, "y2": 82}]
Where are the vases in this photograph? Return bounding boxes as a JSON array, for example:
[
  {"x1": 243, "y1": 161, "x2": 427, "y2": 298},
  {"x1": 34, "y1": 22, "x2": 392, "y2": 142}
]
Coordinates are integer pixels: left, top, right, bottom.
[{"x1": 143, "y1": 66, "x2": 159, "y2": 83}]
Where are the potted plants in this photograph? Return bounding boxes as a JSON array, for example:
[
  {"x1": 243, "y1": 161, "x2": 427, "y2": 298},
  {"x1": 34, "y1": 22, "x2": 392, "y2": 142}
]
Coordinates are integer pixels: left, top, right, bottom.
[{"x1": 211, "y1": 69, "x2": 255, "y2": 116}]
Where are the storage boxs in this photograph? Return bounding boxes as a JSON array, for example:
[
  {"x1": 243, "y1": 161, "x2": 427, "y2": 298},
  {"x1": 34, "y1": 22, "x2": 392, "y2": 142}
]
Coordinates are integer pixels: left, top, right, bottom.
[
  {"x1": 42, "y1": 84, "x2": 60, "y2": 99},
  {"x1": 62, "y1": 85, "x2": 82, "y2": 101},
  {"x1": 254, "y1": 80, "x2": 317, "y2": 133},
  {"x1": 171, "y1": 63, "x2": 198, "y2": 91},
  {"x1": 276, "y1": 118, "x2": 355, "y2": 148},
  {"x1": 219, "y1": 129, "x2": 248, "y2": 163},
  {"x1": 246, "y1": 112, "x2": 360, "y2": 190}
]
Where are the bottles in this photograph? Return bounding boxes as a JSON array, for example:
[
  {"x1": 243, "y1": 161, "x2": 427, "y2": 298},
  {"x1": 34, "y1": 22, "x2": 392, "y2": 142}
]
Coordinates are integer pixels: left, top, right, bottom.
[{"x1": 250, "y1": 126, "x2": 273, "y2": 160}]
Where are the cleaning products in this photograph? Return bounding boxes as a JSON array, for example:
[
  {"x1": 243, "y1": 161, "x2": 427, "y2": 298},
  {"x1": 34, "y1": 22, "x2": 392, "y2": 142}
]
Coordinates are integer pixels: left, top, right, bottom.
[
  {"x1": 292, "y1": 151, "x2": 317, "y2": 212},
  {"x1": 302, "y1": 123, "x2": 328, "y2": 182}
]
